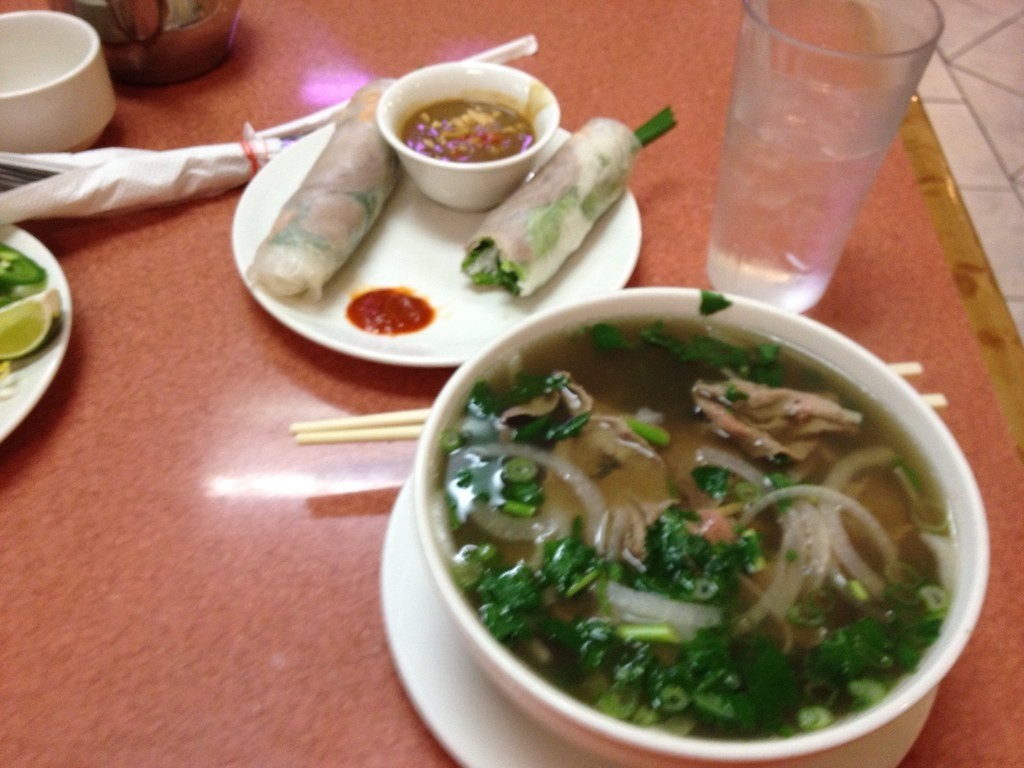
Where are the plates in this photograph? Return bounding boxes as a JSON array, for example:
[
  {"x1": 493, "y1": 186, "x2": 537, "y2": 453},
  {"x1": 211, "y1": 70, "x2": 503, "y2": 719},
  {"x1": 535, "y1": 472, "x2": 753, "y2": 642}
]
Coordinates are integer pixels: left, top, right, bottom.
[
  {"x1": 378, "y1": 473, "x2": 938, "y2": 768},
  {"x1": 232, "y1": 120, "x2": 643, "y2": 366},
  {"x1": 0, "y1": 223, "x2": 72, "y2": 440}
]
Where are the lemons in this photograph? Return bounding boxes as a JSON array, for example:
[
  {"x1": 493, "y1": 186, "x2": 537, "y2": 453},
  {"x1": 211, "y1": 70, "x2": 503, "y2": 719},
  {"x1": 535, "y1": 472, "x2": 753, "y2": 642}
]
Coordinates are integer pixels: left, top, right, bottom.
[{"x1": 0, "y1": 288, "x2": 63, "y2": 361}]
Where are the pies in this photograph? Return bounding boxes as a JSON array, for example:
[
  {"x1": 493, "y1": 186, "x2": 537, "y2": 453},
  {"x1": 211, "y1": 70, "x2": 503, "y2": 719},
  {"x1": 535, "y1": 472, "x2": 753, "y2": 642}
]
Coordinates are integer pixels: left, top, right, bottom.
[
  {"x1": 461, "y1": 105, "x2": 677, "y2": 296},
  {"x1": 246, "y1": 78, "x2": 399, "y2": 303}
]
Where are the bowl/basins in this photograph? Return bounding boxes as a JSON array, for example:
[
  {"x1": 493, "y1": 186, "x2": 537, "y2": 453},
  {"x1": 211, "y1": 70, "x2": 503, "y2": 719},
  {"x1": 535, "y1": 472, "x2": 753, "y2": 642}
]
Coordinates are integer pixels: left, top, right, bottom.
[
  {"x1": 414, "y1": 286, "x2": 989, "y2": 763},
  {"x1": 375, "y1": 62, "x2": 560, "y2": 211},
  {"x1": 0, "y1": 10, "x2": 116, "y2": 153}
]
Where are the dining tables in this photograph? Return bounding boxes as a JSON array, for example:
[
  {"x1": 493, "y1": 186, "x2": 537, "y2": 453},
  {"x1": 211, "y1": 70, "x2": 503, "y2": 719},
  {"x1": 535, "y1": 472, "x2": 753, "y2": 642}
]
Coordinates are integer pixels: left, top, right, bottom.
[{"x1": 0, "y1": 0, "x2": 1024, "y2": 767}]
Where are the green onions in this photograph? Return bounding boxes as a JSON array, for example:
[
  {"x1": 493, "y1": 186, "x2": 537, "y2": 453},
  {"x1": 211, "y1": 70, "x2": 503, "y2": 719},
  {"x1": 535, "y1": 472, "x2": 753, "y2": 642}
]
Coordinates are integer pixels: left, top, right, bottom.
[{"x1": 432, "y1": 287, "x2": 951, "y2": 741}]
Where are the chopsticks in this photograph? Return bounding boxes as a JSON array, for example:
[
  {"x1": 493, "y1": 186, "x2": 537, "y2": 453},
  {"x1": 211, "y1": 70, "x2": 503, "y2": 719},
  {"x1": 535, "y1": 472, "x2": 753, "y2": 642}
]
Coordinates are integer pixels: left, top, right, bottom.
[{"x1": 290, "y1": 363, "x2": 947, "y2": 445}]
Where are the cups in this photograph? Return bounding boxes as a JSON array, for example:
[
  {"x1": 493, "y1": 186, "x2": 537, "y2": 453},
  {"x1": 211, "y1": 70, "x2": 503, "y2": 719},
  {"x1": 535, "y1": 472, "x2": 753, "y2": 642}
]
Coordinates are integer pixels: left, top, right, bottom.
[{"x1": 705, "y1": 0, "x2": 946, "y2": 308}]
